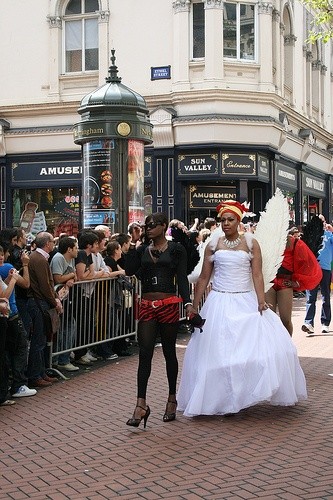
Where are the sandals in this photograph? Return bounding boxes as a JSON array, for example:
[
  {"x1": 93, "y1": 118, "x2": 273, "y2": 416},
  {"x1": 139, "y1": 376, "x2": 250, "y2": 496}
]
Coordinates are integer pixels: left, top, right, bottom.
[{"x1": 0, "y1": 399, "x2": 16, "y2": 405}]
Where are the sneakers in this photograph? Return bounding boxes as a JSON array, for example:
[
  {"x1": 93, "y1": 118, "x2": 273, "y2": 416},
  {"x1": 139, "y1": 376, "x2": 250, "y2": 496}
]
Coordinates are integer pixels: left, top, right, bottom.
[
  {"x1": 321, "y1": 325, "x2": 329, "y2": 333},
  {"x1": 302, "y1": 324, "x2": 314, "y2": 333},
  {"x1": 12, "y1": 384, "x2": 37, "y2": 397}
]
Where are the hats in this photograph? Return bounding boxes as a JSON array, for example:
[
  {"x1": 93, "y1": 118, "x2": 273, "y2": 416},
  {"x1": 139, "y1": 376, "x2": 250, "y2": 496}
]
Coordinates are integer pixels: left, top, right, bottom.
[{"x1": 216, "y1": 199, "x2": 257, "y2": 223}]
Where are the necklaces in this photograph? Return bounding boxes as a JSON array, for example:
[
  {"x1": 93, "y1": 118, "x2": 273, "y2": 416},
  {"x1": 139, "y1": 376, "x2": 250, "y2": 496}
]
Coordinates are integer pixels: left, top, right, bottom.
[{"x1": 223, "y1": 234, "x2": 241, "y2": 249}]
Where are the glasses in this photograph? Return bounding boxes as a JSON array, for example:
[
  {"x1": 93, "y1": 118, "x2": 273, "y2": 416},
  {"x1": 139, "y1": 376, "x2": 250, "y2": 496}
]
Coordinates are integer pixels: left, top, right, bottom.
[
  {"x1": 49, "y1": 241, "x2": 55, "y2": 244},
  {"x1": 144, "y1": 221, "x2": 161, "y2": 230}
]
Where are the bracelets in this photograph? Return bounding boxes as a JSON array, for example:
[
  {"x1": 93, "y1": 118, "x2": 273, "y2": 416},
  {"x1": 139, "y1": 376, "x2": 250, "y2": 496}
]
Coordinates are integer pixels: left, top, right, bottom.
[
  {"x1": 129, "y1": 242, "x2": 136, "y2": 246},
  {"x1": 22, "y1": 264, "x2": 28, "y2": 266},
  {"x1": 296, "y1": 280, "x2": 300, "y2": 288},
  {"x1": 184, "y1": 302, "x2": 193, "y2": 309}
]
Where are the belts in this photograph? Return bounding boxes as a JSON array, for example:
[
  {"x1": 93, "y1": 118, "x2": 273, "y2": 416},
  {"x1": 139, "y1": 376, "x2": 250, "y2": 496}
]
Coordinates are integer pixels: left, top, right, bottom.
[
  {"x1": 8, "y1": 314, "x2": 18, "y2": 321},
  {"x1": 141, "y1": 276, "x2": 174, "y2": 286},
  {"x1": 141, "y1": 295, "x2": 183, "y2": 309}
]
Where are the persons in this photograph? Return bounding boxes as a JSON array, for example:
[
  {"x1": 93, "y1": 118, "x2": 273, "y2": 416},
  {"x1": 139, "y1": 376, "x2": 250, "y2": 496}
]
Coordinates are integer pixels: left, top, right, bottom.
[
  {"x1": 177, "y1": 187, "x2": 307, "y2": 416},
  {"x1": 125, "y1": 211, "x2": 196, "y2": 429},
  {"x1": 0, "y1": 214, "x2": 333, "y2": 406},
  {"x1": 302, "y1": 214, "x2": 333, "y2": 334},
  {"x1": 264, "y1": 215, "x2": 326, "y2": 337}
]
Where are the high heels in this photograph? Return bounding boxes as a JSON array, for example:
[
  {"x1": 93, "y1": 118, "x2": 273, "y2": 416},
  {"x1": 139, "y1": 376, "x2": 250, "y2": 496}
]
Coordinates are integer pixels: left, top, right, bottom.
[
  {"x1": 126, "y1": 404, "x2": 150, "y2": 428},
  {"x1": 163, "y1": 399, "x2": 178, "y2": 422}
]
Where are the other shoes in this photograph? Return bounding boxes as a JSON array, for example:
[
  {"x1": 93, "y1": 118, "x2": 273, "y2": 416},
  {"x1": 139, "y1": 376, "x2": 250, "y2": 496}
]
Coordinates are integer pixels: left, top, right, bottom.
[
  {"x1": 177, "y1": 326, "x2": 188, "y2": 334},
  {"x1": 107, "y1": 353, "x2": 118, "y2": 359},
  {"x1": 56, "y1": 361, "x2": 79, "y2": 371},
  {"x1": 44, "y1": 376, "x2": 58, "y2": 382},
  {"x1": 29, "y1": 379, "x2": 52, "y2": 387},
  {"x1": 82, "y1": 352, "x2": 98, "y2": 361}
]
